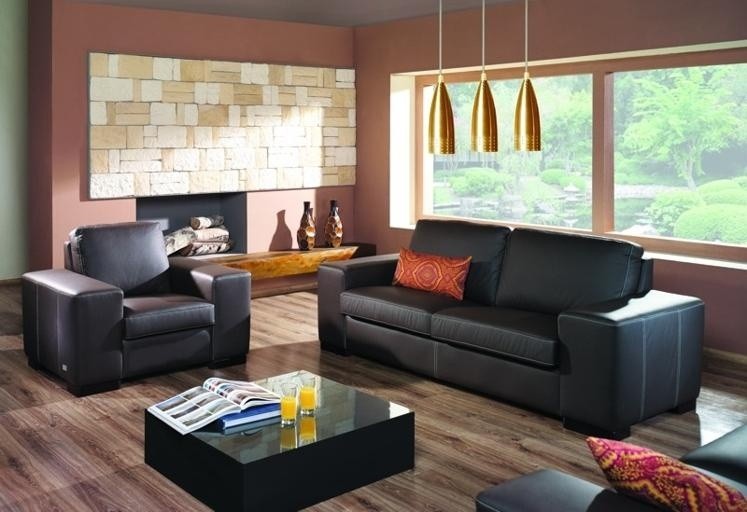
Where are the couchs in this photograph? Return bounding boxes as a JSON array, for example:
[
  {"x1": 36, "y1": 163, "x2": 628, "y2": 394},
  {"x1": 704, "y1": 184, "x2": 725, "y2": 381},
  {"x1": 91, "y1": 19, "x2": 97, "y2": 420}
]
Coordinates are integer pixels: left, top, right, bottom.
[{"x1": 318, "y1": 220, "x2": 705, "y2": 441}]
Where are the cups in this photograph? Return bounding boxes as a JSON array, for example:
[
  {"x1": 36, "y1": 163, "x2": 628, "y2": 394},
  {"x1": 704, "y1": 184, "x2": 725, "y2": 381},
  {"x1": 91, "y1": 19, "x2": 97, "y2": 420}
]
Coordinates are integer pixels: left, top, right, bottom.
[
  {"x1": 284, "y1": 375, "x2": 317, "y2": 429},
  {"x1": 281, "y1": 418, "x2": 317, "y2": 451}
]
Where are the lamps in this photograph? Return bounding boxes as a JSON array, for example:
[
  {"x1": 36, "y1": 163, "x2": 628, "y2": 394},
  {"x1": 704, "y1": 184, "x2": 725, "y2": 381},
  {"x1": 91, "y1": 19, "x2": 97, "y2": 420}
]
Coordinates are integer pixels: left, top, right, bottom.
[
  {"x1": 470, "y1": 1, "x2": 498, "y2": 153},
  {"x1": 513, "y1": 1, "x2": 541, "y2": 152},
  {"x1": 428, "y1": 1, "x2": 456, "y2": 154}
]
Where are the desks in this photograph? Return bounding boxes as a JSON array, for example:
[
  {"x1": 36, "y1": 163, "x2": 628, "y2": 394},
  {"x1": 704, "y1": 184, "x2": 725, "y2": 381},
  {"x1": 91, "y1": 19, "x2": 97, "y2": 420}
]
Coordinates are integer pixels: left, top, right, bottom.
[{"x1": 189, "y1": 243, "x2": 376, "y2": 299}]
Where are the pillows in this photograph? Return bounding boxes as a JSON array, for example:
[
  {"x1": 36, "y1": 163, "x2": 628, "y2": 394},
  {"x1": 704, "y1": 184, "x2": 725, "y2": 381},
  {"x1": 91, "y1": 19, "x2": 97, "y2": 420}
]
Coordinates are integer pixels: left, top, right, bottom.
[
  {"x1": 68, "y1": 222, "x2": 179, "y2": 297},
  {"x1": 586, "y1": 437, "x2": 747, "y2": 512},
  {"x1": 409, "y1": 219, "x2": 512, "y2": 306},
  {"x1": 495, "y1": 227, "x2": 644, "y2": 316},
  {"x1": 391, "y1": 246, "x2": 472, "y2": 301}
]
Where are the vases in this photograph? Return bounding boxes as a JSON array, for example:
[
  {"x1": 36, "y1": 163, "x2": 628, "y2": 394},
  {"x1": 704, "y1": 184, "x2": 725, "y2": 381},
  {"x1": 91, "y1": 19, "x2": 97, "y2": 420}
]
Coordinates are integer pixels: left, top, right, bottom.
[
  {"x1": 324, "y1": 200, "x2": 344, "y2": 248},
  {"x1": 297, "y1": 202, "x2": 317, "y2": 251}
]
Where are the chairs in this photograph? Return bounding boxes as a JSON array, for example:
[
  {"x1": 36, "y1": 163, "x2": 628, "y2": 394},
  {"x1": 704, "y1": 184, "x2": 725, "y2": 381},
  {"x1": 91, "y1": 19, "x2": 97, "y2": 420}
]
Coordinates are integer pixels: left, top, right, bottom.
[
  {"x1": 475, "y1": 423, "x2": 747, "y2": 512},
  {"x1": 21, "y1": 222, "x2": 251, "y2": 398}
]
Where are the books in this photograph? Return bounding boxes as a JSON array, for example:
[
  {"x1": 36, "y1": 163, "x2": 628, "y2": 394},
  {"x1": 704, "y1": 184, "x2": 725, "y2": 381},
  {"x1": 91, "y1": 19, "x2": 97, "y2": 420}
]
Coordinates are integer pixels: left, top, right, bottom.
[
  {"x1": 216, "y1": 401, "x2": 282, "y2": 431},
  {"x1": 145, "y1": 375, "x2": 284, "y2": 439},
  {"x1": 216, "y1": 416, "x2": 282, "y2": 435}
]
